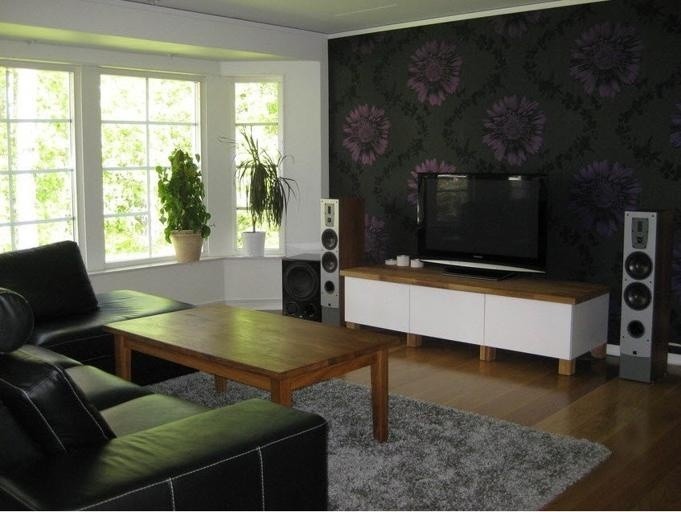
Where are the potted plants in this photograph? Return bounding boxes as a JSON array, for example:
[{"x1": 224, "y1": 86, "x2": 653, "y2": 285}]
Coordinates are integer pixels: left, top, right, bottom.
[
  {"x1": 155, "y1": 144, "x2": 214, "y2": 264},
  {"x1": 234, "y1": 123, "x2": 299, "y2": 259}
]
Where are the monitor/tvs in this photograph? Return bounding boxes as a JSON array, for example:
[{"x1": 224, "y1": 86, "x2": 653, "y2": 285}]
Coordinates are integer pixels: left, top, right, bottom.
[{"x1": 420, "y1": 174, "x2": 547, "y2": 280}]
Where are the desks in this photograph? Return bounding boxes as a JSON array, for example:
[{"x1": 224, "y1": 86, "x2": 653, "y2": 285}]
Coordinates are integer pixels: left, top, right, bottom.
[{"x1": 337, "y1": 262, "x2": 612, "y2": 378}]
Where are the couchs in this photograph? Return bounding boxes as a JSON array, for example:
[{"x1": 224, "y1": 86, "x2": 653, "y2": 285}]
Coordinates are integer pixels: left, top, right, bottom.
[
  {"x1": 0, "y1": 239, "x2": 198, "y2": 388},
  {"x1": 0, "y1": 286, "x2": 330, "y2": 512}
]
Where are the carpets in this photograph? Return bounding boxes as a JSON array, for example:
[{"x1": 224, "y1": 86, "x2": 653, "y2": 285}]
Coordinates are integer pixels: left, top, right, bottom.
[{"x1": 145, "y1": 370, "x2": 612, "y2": 512}]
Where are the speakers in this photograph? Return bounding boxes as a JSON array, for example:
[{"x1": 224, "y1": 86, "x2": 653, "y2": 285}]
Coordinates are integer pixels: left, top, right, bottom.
[
  {"x1": 320, "y1": 199, "x2": 342, "y2": 324},
  {"x1": 618, "y1": 211, "x2": 670, "y2": 382},
  {"x1": 281, "y1": 253, "x2": 320, "y2": 322}
]
